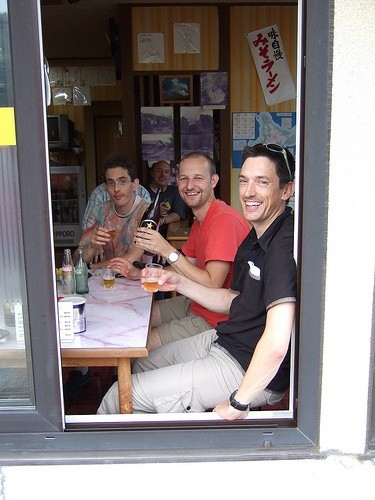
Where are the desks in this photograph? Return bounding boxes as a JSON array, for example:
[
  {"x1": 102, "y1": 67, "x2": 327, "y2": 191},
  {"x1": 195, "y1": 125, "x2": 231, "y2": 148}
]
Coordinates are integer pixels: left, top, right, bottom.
[
  {"x1": 0, "y1": 269, "x2": 154, "y2": 415},
  {"x1": 166, "y1": 220, "x2": 190, "y2": 297}
]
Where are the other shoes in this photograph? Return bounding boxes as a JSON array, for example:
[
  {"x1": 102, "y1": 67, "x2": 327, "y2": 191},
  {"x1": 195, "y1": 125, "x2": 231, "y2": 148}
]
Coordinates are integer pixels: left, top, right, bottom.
[
  {"x1": 63, "y1": 369, "x2": 91, "y2": 397},
  {"x1": 112, "y1": 375, "x2": 117, "y2": 384}
]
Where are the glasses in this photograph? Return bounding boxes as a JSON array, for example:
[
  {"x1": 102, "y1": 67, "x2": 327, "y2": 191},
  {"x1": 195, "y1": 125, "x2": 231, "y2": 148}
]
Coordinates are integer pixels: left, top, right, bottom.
[
  {"x1": 253, "y1": 143, "x2": 292, "y2": 177},
  {"x1": 106, "y1": 179, "x2": 131, "y2": 187}
]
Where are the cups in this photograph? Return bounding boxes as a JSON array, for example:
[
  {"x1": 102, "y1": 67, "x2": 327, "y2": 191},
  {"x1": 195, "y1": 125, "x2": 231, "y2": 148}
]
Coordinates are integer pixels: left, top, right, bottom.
[
  {"x1": 102, "y1": 266, "x2": 115, "y2": 288},
  {"x1": 144, "y1": 263, "x2": 163, "y2": 293},
  {"x1": 104, "y1": 221, "x2": 116, "y2": 240}
]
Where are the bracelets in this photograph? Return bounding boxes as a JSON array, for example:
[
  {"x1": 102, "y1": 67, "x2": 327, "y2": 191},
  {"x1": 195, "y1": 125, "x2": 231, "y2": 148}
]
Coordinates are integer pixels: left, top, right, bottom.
[{"x1": 87, "y1": 264, "x2": 91, "y2": 269}]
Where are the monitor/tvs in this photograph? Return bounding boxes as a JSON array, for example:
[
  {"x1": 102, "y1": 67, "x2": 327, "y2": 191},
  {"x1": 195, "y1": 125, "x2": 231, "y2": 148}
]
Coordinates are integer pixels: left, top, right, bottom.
[{"x1": 46, "y1": 114, "x2": 68, "y2": 149}]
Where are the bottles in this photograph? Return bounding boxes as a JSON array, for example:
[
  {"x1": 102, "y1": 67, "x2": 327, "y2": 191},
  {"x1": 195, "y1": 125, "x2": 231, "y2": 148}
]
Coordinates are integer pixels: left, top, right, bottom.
[
  {"x1": 133, "y1": 187, "x2": 164, "y2": 244},
  {"x1": 61, "y1": 248, "x2": 75, "y2": 295},
  {"x1": 57, "y1": 202, "x2": 78, "y2": 223},
  {"x1": 75, "y1": 249, "x2": 89, "y2": 295},
  {"x1": 4, "y1": 300, "x2": 20, "y2": 328},
  {"x1": 59, "y1": 297, "x2": 87, "y2": 334}
]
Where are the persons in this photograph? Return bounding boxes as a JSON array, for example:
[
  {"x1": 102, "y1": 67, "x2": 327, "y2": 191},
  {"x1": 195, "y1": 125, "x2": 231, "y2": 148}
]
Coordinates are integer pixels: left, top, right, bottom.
[
  {"x1": 82, "y1": 183, "x2": 151, "y2": 231},
  {"x1": 63, "y1": 159, "x2": 152, "y2": 400},
  {"x1": 98, "y1": 152, "x2": 253, "y2": 397},
  {"x1": 145, "y1": 160, "x2": 190, "y2": 269},
  {"x1": 96, "y1": 144, "x2": 298, "y2": 421}
]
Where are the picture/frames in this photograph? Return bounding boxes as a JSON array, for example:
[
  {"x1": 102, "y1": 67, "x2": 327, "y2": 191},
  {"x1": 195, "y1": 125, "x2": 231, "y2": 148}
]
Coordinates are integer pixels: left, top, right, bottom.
[{"x1": 159, "y1": 75, "x2": 193, "y2": 103}]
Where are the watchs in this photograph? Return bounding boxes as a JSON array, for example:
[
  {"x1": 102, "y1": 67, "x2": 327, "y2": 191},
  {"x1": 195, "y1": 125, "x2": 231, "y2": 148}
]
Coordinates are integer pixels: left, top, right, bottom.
[
  {"x1": 229, "y1": 390, "x2": 249, "y2": 412},
  {"x1": 163, "y1": 218, "x2": 166, "y2": 224},
  {"x1": 167, "y1": 250, "x2": 181, "y2": 265}
]
